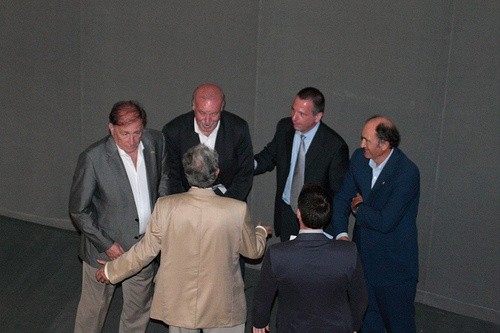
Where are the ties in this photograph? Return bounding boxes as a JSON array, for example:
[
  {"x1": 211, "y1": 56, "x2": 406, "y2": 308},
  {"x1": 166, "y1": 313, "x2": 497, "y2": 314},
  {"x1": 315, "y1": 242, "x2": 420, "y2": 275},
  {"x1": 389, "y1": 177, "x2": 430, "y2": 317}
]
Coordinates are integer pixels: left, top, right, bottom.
[{"x1": 290, "y1": 134, "x2": 306, "y2": 214}]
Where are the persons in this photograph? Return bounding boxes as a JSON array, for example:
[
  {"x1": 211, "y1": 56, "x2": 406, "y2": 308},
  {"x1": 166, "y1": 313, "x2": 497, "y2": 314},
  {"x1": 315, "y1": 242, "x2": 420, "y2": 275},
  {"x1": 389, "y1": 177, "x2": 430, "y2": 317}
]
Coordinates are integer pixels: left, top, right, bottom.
[
  {"x1": 330, "y1": 114, "x2": 419, "y2": 333},
  {"x1": 94, "y1": 144, "x2": 273, "y2": 333},
  {"x1": 252, "y1": 185, "x2": 370, "y2": 332},
  {"x1": 65, "y1": 83, "x2": 350, "y2": 333}
]
